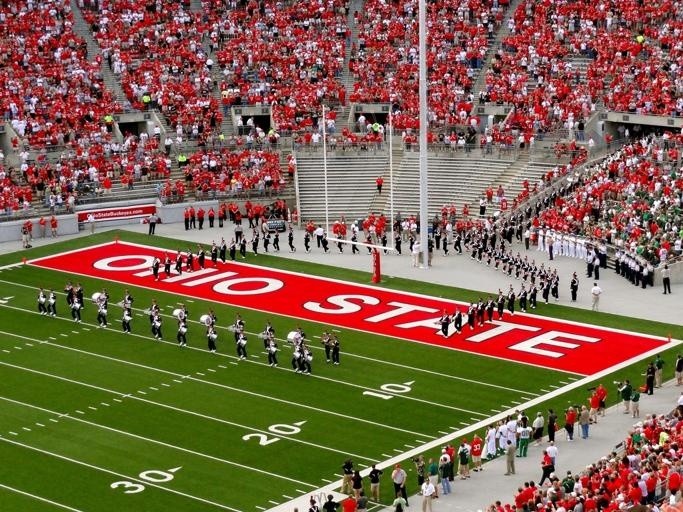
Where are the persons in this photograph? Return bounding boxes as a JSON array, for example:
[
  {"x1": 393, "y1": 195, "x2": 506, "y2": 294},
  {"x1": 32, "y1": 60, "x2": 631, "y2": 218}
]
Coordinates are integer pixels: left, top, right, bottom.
[
  {"x1": 293, "y1": 352, "x2": 682, "y2": 512},
  {"x1": 1, "y1": 0, "x2": 683, "y2": 339},
  {"x1": 206, "y1": 324, "x2": 340, "y2": 375}
]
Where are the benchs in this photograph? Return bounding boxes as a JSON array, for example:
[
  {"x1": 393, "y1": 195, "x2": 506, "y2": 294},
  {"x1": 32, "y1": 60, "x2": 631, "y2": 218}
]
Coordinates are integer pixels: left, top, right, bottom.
[{"x1": 0, "y1": 0, "x2": 681, "y2": 293}]
